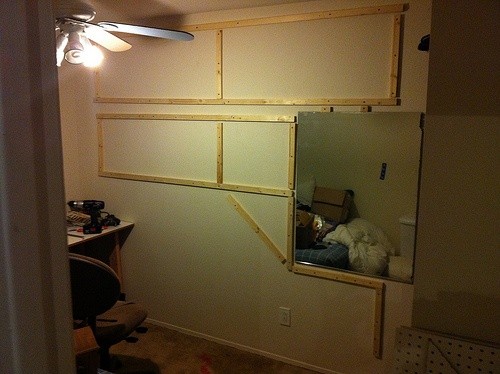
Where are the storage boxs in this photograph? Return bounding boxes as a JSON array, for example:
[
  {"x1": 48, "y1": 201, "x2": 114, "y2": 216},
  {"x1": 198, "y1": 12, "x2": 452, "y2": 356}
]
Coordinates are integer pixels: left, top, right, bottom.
[
  {"x1": 295, "y1": 209, "x2": 314, "y2": 248},
  {"x1": 310, "y1": 187, "x2": 351, "y2": 224}
]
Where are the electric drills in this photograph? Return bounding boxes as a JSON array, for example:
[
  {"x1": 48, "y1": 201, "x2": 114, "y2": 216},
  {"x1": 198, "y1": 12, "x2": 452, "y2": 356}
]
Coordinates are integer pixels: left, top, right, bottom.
[{"x1": 67, "y1": 200, "x2": 106, "y2": 233}]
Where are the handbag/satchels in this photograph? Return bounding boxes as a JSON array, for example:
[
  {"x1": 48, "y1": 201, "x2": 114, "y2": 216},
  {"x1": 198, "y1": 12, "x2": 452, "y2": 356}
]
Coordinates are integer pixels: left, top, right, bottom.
[{"x1": 311, "y1": 186, "x2": 349, "y2": 223}]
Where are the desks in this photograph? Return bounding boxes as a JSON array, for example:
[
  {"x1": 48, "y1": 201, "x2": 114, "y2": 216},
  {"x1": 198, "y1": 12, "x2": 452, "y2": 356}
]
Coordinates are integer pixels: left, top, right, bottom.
[
  {"x1": 67, "y1": 213, "x2": 135, "y2": 301},
  {"x1": 296, "y1": 238, "x2": 348, "y2": 270}
]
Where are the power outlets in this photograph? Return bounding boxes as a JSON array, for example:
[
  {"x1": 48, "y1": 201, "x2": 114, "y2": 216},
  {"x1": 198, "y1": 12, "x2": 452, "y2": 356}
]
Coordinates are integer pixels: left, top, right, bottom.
[{"x1": 279, "y1": 307, "x2": 290, "y2": 326}]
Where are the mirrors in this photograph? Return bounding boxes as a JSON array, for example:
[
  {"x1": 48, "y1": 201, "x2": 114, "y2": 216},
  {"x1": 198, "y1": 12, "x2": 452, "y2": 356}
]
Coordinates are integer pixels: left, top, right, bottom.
[{"x1": 294, "y1": 105, "x2": 425, "y2": 284}]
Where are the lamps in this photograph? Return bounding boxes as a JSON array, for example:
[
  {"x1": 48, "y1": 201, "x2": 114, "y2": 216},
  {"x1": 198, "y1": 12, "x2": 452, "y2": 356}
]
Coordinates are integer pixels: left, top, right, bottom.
[{"x1": 65, "y1": 29, "x2": 93, "y2": 65}]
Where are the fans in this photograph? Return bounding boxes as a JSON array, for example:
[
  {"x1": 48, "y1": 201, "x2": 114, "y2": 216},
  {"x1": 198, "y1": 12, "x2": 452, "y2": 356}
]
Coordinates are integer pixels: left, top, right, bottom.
[{"x1": 52, "y1": 5, "x2": 194, "y2": 68}]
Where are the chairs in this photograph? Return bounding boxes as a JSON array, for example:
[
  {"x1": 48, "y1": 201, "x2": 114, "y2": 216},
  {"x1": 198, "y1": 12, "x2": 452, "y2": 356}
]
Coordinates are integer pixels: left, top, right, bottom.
[{"x1": 67, "y1": 252, "x2": 150, "y2": 371}]
